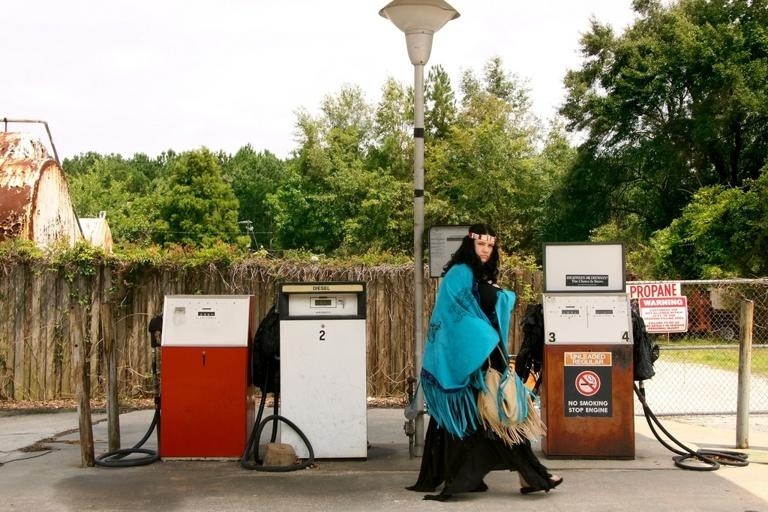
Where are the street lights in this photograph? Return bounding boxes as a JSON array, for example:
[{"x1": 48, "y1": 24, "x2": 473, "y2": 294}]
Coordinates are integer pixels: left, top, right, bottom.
[{"x1": 379, "y1": 0, "x2": 461, "y2": 455}]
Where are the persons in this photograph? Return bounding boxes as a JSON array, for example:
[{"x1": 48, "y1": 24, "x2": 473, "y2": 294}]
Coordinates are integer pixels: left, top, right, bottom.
[{"x1": 404, "y1": 222, "x2": 563, "y2": 494}]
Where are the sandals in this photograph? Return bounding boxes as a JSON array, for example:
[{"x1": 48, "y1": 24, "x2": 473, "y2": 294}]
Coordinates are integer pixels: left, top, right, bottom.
[
  {"x1": 444, "y1": 481, "x2": 489, "y2": 493},
  {"x1": 519, "y1": 475, "x2": 565, "y2": 495}
]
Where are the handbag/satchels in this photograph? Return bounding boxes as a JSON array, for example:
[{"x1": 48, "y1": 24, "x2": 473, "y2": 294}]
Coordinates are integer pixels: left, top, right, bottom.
[{"x1": 476, "y1": 367, "x2": 534, "y2": 427}]
